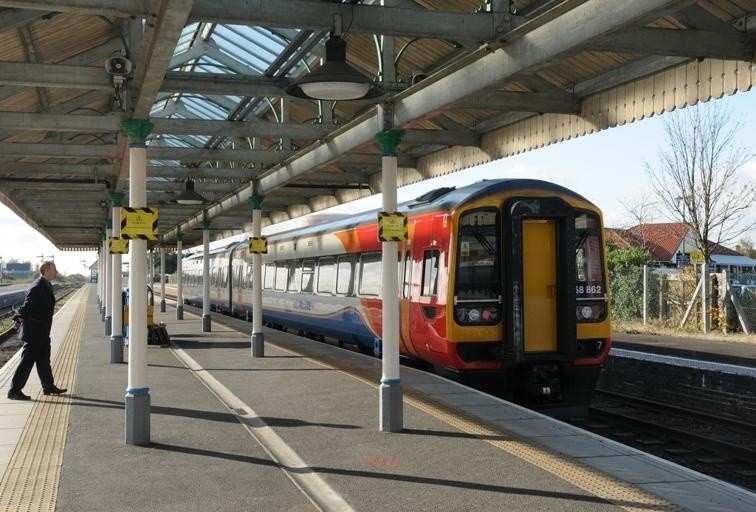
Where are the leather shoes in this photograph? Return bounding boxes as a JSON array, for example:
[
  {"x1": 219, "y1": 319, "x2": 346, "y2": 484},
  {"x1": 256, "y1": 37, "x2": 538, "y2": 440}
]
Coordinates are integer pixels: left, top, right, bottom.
[
  {"x1": 44, "y1": 386, "x2": 68, "y2": 395},
  {"x1": 7, "y1": 390, "x2": 30, "y2": 400}
]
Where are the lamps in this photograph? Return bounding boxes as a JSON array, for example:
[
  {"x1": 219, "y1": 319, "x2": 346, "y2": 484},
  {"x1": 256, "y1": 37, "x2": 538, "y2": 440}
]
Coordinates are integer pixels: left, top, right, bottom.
[
  {"x1": 172, "y1": 175, "x2": 209, "y2": 206},
  {"x1": 280, "y1": 12, "x2": 376, "y2": 101}
]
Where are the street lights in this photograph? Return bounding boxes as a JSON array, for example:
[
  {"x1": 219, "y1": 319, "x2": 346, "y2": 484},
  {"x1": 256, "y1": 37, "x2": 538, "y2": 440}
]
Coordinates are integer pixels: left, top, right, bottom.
[{"x1": 674, "y1": 191, "x2": 693, "y2": 308}]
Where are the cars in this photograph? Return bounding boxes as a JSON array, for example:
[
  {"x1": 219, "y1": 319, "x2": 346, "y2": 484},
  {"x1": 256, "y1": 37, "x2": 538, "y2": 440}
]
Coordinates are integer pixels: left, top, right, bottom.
[{"x1": 730, "y1": 276, "x2": 756, "y2": 296}]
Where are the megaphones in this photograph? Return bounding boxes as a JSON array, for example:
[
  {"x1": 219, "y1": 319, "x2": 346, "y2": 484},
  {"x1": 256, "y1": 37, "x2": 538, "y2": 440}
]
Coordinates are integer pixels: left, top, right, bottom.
[{"x1": 103, "y1": 48, "x2": 133, "y2": 76}]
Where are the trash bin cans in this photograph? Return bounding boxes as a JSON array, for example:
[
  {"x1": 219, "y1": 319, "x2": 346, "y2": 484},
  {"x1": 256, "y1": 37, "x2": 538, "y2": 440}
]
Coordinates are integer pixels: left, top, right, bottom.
[{"x1": 124, "y1": 285, "x2": 154, "y2": 346}]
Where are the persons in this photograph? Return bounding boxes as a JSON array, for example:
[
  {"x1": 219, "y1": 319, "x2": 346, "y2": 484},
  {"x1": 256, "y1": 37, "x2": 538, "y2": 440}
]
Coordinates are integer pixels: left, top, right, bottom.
[{"x1": 7, "y1": 261, "x2": 68, "y2": 400}]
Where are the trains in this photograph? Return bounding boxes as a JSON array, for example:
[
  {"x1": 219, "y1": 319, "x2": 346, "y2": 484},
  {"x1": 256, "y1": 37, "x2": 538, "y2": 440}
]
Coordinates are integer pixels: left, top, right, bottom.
[{"x1": 153, "y1": 176, "x2": 610, "y2": 408}]
